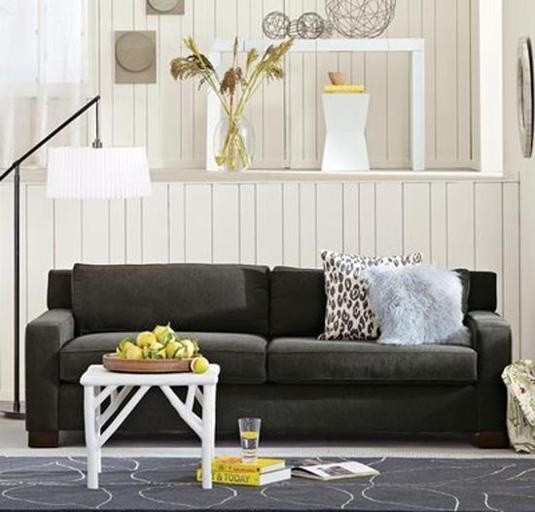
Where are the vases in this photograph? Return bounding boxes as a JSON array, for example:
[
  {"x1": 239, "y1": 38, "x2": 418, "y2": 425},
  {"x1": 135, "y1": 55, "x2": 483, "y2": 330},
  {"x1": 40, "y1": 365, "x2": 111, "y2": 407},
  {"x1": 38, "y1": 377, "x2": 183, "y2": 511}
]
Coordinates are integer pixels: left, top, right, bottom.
[{"x1": 213, "y1": 115, "x2": 256, "y2": 173}]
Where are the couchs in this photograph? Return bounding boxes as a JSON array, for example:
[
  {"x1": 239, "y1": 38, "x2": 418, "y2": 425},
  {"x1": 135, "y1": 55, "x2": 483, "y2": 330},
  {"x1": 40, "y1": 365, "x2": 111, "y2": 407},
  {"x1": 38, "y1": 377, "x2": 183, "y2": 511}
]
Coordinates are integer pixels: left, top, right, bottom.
[{"x1": 25, "y1": 264, "x2": 513, "y2": 449}]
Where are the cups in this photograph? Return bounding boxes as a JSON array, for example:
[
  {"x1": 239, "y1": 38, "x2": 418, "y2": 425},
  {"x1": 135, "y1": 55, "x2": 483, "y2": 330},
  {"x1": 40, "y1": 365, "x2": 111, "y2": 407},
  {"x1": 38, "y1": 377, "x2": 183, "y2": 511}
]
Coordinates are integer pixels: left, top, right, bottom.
[{"x1": 237, "y1": 417, "x2": 263, "y2": 464}]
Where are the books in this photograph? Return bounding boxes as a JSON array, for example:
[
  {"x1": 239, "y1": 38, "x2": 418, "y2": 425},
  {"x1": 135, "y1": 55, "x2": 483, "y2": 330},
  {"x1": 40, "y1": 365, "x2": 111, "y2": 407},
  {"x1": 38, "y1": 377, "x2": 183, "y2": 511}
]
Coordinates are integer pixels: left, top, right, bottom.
[
  {"x1": 196, "y1": 456, "x2": 291, "y2": 487},
  {"x1": 291, "y1": 460, "x2": 381, "y2": 481}
]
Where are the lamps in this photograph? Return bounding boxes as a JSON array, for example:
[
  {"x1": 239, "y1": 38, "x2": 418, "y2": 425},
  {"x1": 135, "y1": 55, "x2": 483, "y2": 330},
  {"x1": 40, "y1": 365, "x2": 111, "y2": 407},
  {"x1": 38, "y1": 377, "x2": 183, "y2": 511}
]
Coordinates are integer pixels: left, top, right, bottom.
[{"x1": 0, "y1": 95, "x2": 153, "y2": 415}]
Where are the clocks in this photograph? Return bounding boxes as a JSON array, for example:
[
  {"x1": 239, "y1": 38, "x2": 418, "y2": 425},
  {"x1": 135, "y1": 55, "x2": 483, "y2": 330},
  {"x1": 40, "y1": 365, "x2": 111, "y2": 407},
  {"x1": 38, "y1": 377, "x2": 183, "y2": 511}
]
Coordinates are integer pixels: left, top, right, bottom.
[{"x1": 515, "y1": 38, "x2": 532, "y2": 158}]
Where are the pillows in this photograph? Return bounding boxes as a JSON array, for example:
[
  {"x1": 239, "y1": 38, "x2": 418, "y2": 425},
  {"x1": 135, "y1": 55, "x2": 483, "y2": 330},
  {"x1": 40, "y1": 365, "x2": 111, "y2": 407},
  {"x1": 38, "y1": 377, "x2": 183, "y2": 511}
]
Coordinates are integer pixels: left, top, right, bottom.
[
  {"x1": 317, "y1": 249, "x2": 423, "y2": 341},
  {"x1": 365, "y1": 266, "x2": 471, "y2": 346}
]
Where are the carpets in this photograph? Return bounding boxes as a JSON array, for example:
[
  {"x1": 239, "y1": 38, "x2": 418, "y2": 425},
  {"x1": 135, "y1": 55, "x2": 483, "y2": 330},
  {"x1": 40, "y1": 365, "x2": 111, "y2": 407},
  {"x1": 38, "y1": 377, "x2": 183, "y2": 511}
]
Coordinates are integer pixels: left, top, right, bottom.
[{"x1": 0, "y1": 457, "x2": 535, "y2": 512}]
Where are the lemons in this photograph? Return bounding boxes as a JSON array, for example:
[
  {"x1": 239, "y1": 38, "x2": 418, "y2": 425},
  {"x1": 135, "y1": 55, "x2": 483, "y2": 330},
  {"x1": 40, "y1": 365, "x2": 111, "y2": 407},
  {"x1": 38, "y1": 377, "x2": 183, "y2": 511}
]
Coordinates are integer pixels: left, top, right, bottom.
[{"x1": 116, "y1": 324, "x2": 210, "y2": 373}]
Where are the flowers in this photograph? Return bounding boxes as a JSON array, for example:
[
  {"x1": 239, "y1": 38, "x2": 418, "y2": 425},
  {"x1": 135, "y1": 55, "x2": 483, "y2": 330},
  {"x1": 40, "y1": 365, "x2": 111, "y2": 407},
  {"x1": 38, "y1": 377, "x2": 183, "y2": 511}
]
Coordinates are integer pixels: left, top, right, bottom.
[{"x1": 169, "y1": 36, "x2": 296, "y2": 115}]
